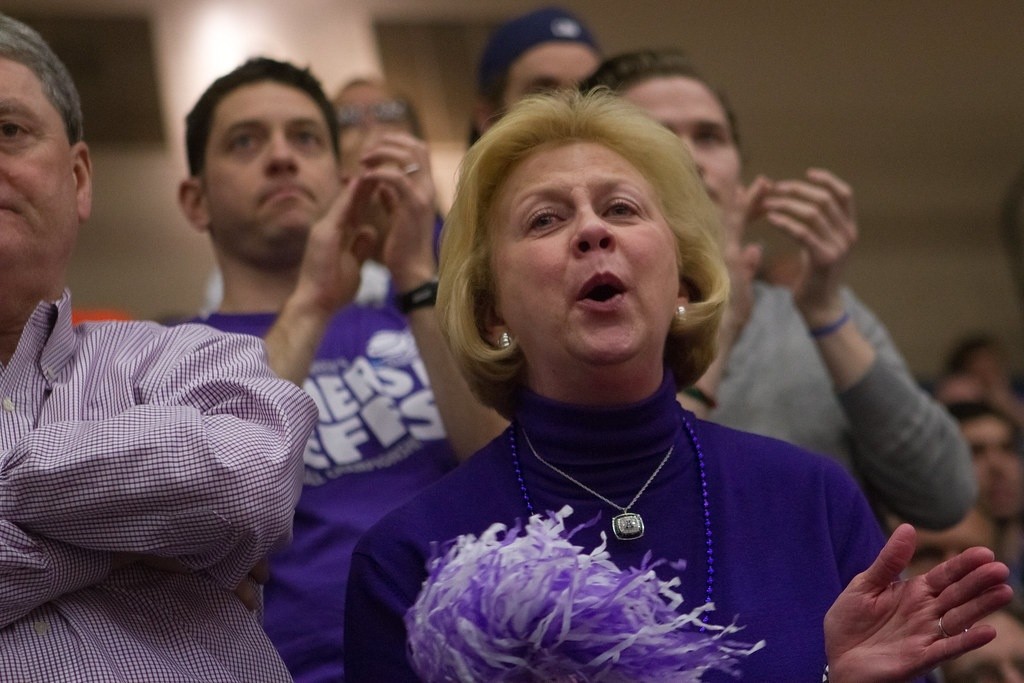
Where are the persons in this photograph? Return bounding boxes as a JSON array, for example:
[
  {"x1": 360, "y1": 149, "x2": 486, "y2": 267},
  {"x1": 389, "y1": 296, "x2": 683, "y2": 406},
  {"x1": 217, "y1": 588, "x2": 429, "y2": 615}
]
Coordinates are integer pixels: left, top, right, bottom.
[
  {"x1": 164, "y1": 56, "x2": 512, "y2": 683},
  {"x1": 330, "y1": 77, "x2": 444, "y2": 309},
  {"x1": 338, "y1": 84, "x2": 1014, "y2": 683},
  {"x1": 470, "y1": 6, "x2": 602, "y2": 145},
  {"x1": 886, "y1": 333, "x2": 1024, "y2": 683},
  {"x1": 581, "y1": 45, "x2": 977, "y2": 533},
  {"x1": 0, "y1": 11, "x2": 320, "y2": 683}
]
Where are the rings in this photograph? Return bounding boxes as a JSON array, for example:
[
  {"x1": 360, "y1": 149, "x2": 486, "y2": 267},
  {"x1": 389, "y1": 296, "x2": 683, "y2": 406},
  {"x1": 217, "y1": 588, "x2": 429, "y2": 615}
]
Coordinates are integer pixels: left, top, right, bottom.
[
  {"x1": 404, "y1": 163, "x2": 418, "y2": 174},
  {"x1": 938, "y1": 617, "x2": 967, "y2": 638}
]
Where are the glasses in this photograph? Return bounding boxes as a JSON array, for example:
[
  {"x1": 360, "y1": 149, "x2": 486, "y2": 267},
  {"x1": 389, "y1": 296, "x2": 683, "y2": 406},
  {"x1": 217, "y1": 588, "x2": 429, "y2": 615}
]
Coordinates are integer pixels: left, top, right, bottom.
[{"x1": 329, "y1": 100, "x2": 416, "y2": 134}]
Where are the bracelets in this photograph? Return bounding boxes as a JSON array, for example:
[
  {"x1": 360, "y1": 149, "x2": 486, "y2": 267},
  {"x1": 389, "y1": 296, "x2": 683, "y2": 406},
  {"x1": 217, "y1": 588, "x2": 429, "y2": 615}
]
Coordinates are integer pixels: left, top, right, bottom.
[
  {"x1": 395, "y1": 282, "x2": 439, "y2": 313},
  {"x1": 809, "y1": 311, "x2": 850, "y2": 337},
  {"x1": 683, "y1": 386, "x2": 717, "y2": 410}
]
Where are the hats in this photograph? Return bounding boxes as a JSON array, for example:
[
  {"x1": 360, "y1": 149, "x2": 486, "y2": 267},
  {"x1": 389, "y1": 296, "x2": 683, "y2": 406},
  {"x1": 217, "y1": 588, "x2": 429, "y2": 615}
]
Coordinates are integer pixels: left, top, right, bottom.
[{"x1": 468, "y1": 9, "x2": 596, "y2": 149}]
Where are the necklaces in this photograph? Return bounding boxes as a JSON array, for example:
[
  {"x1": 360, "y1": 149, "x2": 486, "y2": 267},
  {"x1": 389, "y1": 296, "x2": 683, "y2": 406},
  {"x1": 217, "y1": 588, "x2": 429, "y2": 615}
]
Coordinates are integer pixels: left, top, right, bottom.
[
  {"x1": 509, "y1": 416, "x2": 714, "y2": 631},
  {"x1": 522, "y1": 427, "x2": 675, "y2": 541}
]
